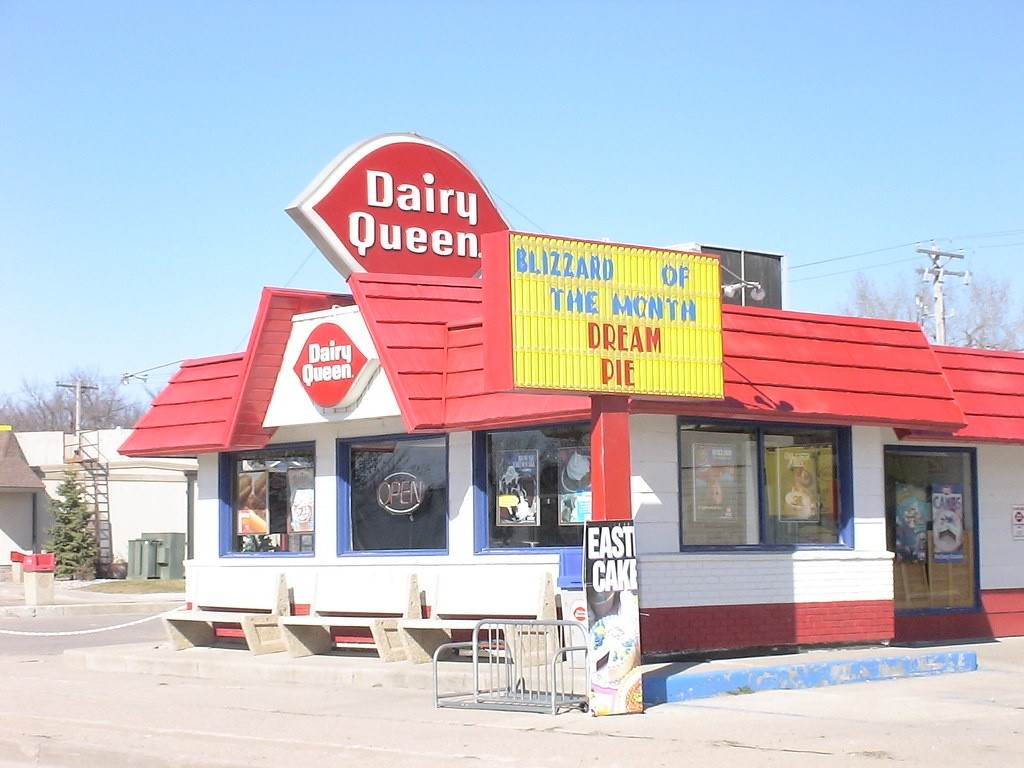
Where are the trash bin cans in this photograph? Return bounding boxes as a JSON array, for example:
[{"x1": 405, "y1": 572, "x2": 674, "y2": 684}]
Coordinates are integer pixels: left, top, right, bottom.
[
  {"x1": 10, "y1": 551, "x2": 29, "y2": 583},
  {"x1": 22, "y1": 553, "x2": 55, "y2": 605},
  {"x1": 556, "y1": 549, "x2": 587, "y2": 669}
]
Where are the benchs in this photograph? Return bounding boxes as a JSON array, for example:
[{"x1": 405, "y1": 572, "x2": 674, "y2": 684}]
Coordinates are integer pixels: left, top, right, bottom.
[
  {"x1": 162, "y1": 574, "x2": 291, "y2": 656},
  {"x1": 398, "y1": 572, "x2": 562, "y2": 669},
  {"x1": 284, "y1": 574, "x2": 423, "y2": 663}
]
